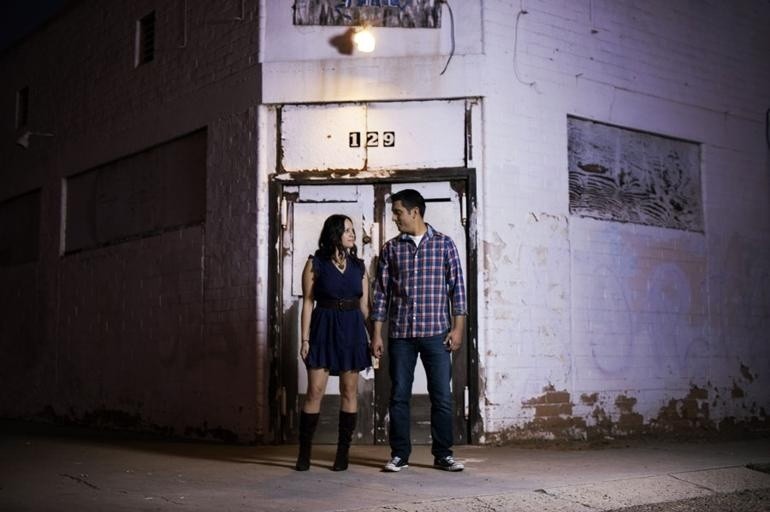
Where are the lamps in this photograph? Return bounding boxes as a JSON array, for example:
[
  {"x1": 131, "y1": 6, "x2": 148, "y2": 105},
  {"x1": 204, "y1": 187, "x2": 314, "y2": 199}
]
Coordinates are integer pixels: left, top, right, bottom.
[{"x1": 350, "y1": 21, "x2": 375, "y2": 52}]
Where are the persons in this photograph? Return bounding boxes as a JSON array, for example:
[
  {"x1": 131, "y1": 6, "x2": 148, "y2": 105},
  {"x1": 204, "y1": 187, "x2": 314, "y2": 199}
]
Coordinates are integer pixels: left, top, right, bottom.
[
  {"x1": 294, "y1": 214, "x2": 380, "y2": 472},
  {"x1": 368, "y1": 188, "x2": 470, "y2": 473}
]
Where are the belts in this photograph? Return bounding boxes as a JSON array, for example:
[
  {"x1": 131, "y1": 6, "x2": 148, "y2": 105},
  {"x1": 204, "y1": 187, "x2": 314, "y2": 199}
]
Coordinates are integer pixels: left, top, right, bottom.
[{"x1": 317, "y1": 298, "x2": 360, "y2": 311}]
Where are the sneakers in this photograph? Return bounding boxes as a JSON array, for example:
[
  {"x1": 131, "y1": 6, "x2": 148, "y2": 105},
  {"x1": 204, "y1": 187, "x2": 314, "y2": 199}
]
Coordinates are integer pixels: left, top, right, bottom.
[
  {"x1": 434, "y1": 455, "x2": 464, "y2": 471},
  {"x1": 383, "y1": 457, "x2": 408, "y2": 472}
]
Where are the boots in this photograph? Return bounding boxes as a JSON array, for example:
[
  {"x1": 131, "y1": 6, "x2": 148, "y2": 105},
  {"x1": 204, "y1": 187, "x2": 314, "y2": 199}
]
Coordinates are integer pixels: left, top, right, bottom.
[
  {"x1": 333, "y1": 410, "x2": 357, "y2": 471},
  {"x1": 296, "y1": 409, "x2": 319, "y2": 471}
]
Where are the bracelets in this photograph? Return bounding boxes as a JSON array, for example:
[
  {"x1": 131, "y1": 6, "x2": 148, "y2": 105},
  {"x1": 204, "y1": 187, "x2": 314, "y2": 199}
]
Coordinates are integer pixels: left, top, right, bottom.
[{"x1": 302, "y1": 339, "x2": 308, "y2": 341}]
